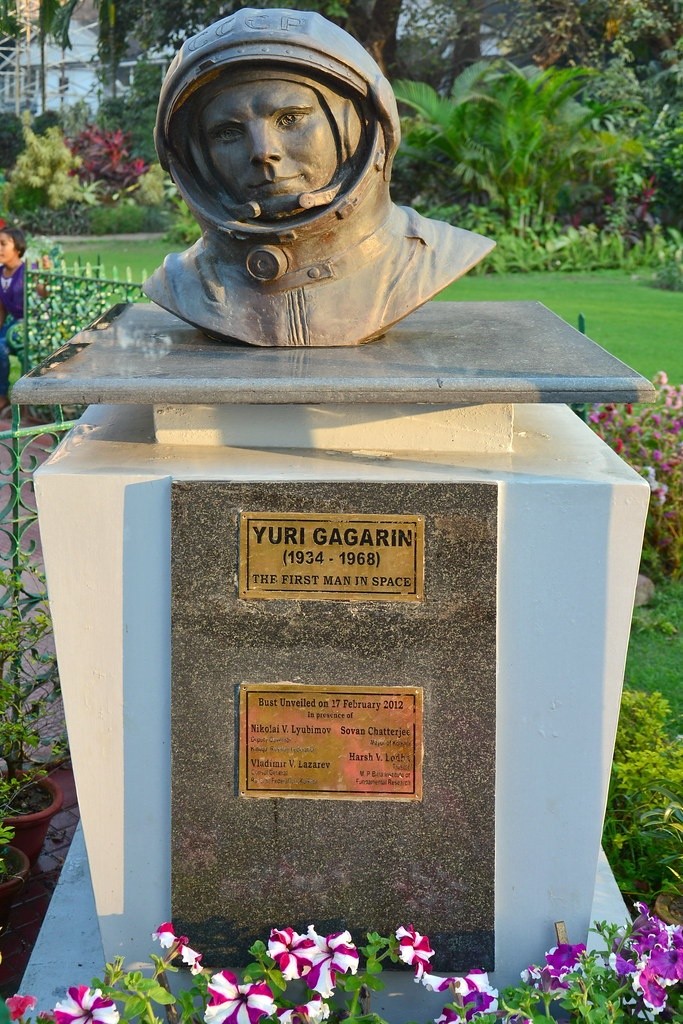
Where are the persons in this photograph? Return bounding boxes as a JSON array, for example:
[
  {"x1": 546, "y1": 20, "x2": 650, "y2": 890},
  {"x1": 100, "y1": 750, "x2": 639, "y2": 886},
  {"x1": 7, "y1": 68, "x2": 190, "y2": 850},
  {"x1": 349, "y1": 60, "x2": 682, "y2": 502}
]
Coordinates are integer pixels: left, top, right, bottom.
[
  {"x1": 1, "y1": 222, "x2": 48, "y2": 414},
  {"x1": 137, "y1": 8, "x2": 498, "y2": 345}
]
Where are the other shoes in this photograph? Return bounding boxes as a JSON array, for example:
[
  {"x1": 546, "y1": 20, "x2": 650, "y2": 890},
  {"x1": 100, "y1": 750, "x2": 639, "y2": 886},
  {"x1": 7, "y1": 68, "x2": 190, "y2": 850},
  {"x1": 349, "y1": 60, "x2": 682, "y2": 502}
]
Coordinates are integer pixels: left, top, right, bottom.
[{"x1": 0, "y1": 401, "x2": 11, "y2": 420}]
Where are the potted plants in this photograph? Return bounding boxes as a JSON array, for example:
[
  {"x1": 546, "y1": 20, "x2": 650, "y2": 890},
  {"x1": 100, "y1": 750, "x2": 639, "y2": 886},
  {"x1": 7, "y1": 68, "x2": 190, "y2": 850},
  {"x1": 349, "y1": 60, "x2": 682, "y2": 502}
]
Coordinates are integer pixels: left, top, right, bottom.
[{"x1": 1, "y1": 543, "x2": 63, "y2": 916}]
